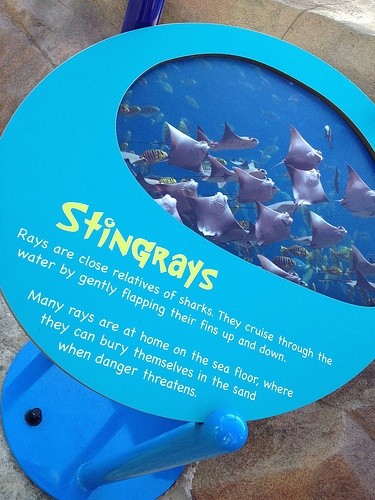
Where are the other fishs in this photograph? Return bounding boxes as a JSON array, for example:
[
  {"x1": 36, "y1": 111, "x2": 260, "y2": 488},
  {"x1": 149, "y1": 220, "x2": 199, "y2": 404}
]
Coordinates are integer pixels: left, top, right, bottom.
[{"x1": 119, "y1": 58, "x2": 375, "y2": 307}]
